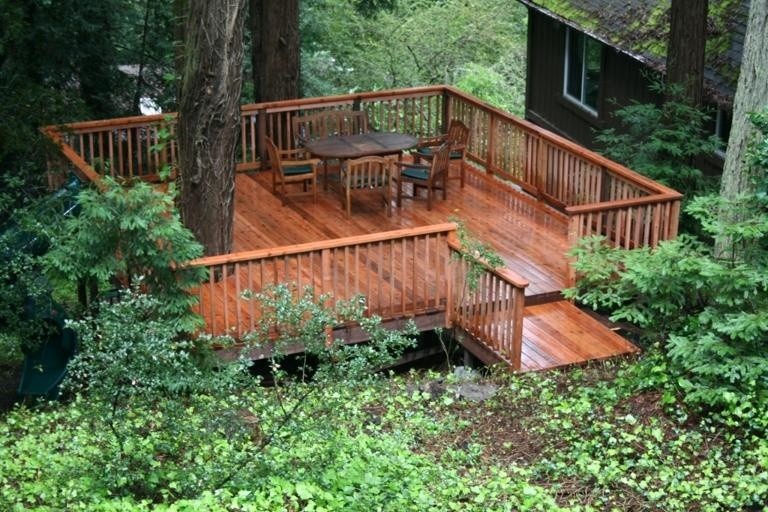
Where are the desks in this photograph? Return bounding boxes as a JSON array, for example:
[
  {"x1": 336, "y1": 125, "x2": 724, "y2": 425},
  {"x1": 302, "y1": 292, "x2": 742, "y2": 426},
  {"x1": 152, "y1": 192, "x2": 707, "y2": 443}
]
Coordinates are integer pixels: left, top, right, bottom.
[{"x1": 303, "y1": 132, "x2": 417, "y2": 190}]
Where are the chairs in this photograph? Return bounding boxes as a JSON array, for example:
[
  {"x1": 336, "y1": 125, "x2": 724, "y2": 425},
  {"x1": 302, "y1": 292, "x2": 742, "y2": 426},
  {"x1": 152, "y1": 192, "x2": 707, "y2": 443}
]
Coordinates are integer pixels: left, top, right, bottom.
[
  {"x1": 292, "y1": 112, "x2": 342, "y2": 185},
  {"x1": 397, "y1": 139, "x2": 453, "y2": 212},
  {"x1": 414, "y1": 119, "x2": 471, "y2": 189},
  {"x1": 263, "y1": 135, "x2": 321, "y2": 207},
  {"x1": 336, "y1": 109, "x2": 367, "y2": 136},
  {"x1": 341, "y1": 155, "x2": 394, "y2": 220}
]
